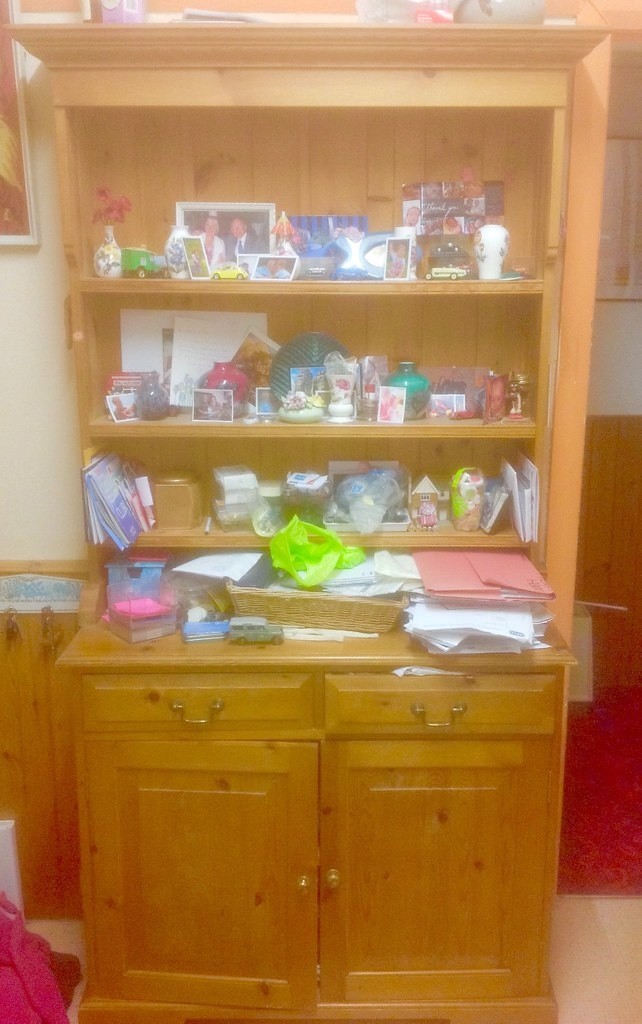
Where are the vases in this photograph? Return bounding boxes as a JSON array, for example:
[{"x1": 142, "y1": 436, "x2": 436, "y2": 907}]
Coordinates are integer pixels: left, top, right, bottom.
[
  {"x1": 393, "y1": 225, "x2": 419, "y2": 279},
  {"x1": 276, "y1": 407, "x2": 325, "y2": 424},
  {"x1": 201, "y1": 360, "x2": 250, "y2": 419},
  {"x1": 94, "y1": 226, "x2": 123, "y2": 276},
  {"x1": 164, "y1": 222, "x2": 192, "y2": 279},
  {"x1": 327, "y1": 370, "x2": 360, "y2": 422},
  {"x1": 472, "y1": 222, "x2": 511, "y2": 279},
  {"x1": 381, "y1": 358, "x2": 425, "y2": 419},
  {"x1": 133, "y1": 370, "x2": 170, "y2": 420},
  {"x1": 272, "y1": 235, "x2": 302, "y2": 280}
]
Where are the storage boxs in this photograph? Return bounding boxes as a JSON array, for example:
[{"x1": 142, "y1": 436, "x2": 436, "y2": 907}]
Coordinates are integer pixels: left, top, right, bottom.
[
  {"x1": 259, "y1": 478, "x2": 285, "y2": 508},
  {"x1": 103, "y1": 578, "x2": 177, "y2": 644},
  {"x1": 419, "y1": 361, "x2": 495, "y2": 416},
  {"x1": 210, "y1": 496, "x2": 252, "y2": 530},
  {"x1": 322, "y1": 505, "x2": 413, "y2": 532},
  {"x1": 103, "y1": 551, "x2": 166, "y2": 601},
  {"x1": 212, "y1": 463, "x2": 259, "y2": 504}
]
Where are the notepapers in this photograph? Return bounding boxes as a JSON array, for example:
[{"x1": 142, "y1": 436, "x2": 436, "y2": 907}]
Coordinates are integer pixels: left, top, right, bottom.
[{"x1": 113, "y1": 598, "x2": 171, "y2": 619}]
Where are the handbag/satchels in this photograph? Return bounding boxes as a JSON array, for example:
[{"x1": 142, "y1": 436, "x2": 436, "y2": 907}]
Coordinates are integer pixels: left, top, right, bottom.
[{"x1": 268, "y1": 515, "x2": 366, "y2": 588}]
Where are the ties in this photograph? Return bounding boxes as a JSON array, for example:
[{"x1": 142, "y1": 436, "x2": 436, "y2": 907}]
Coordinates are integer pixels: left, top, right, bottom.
[{"x1": 237, "y1": 241, "x2": 245, "y2": 254}]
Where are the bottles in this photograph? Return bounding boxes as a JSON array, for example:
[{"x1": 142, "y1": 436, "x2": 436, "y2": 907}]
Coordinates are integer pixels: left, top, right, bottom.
[
  {"x1": 364, "y1": 385, "x2": 376, "y2": 421},
  {"x1": 385, "y1": 362, "x2": 430, "y2": 420},
  {"x1": 165, "y1": 226, "x2": 189, "y2": 279},
  {"x1": 507, "y1": 374, "x2": 530, "y2": 418},
  {"x1": 137, "y1": 373, "x2": 169, "y2": 421},
  {"x1": 474, "y1": 224, "x2": 510, "y2": 280},
  {"x1": 259, "y1": 480, "x2": 281, "y2": 510},
  {"x1": 202, "y1": 362, "x2": 249, "y2": 417}
]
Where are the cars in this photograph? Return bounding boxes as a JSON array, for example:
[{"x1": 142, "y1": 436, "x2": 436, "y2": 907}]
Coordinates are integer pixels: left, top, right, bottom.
[
  {"x1": 212, "y1": 264, "x2": 248, "y2": 280},
  {"x1": 425, "y1": 262, "x2": 469, "y2": 280}
]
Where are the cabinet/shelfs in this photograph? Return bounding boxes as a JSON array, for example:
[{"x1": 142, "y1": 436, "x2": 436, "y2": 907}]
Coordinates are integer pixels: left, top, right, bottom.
[
  {"x1": 21, "y1": 22, "x2": 617, "y2": 624},
  {"x1": 54, "y1": 610, "x2": 584, "y2": 1024}
]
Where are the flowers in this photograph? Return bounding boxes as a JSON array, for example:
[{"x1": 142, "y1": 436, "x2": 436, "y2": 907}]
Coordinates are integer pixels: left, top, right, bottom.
[
  {"x1": 280, "y1": 388, "x2": 326, "y2": 409},
  {"x1": 89, "y1": 184, "x2": 136, "y2": 225}
]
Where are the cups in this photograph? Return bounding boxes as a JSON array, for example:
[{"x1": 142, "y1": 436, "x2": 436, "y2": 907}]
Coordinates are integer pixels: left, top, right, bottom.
[{"x1": 450, "y1": 473, "x2": 486, "y2": 530}]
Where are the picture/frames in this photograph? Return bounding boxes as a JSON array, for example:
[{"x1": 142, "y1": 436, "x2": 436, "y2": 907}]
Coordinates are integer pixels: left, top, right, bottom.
[
  {"x1": 0, "y1": 0, "x2": 46, "y2": 248},
  {"x1": 381, "y1": 236, "x2": 411, "y2": 281},
  {"x1": 429, "y1": 392, "x2": 456, "y2": 417},
  {"x1": 255, "y1": 386, "x2": 276, "y2": 415},
  {"x1": 236, "y1": 253, "x2": 269, "y2": 278},
  {"x1": 181, "y1": 236, "x2": 211, "y2": 279},
  {"x1": 376, "y1": 385, "x2": 408, "y2": 424},
  {"x1": 250, "y1": 253, "x2": 300, "y2": 281},
  {"x1": 174, "y1": 201, "x2": 279, "y2": 274},
  {"x1": 456, "y1": 393, "x2": 466, "y2": 413}
]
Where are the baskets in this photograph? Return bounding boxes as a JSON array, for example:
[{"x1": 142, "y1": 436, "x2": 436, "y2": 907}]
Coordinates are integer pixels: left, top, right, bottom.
[{"x1": 226, "y1": 554, "x2": 409, "y2": 635}]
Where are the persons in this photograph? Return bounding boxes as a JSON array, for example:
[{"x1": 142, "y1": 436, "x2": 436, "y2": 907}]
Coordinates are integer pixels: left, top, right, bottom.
[
  {"x1": 447, "y1": 218, "x2": 461, "y2": 233},
  {"x1": 200, "y1": 216, "x2": 224, "y2": 267},
  {"x1": 432, "y1": 399, "x2": 447, "y2": 415},
  {"x1": 390, "y1": 243, "x2": 406, "y2": 266},
  {"x1": 199, "y1": 396, "x2": 221, "y2": 419},
  {"x1": 380, "y1": 389, "x2": 404, "y2": 423},
  {"x1": 405, "y1": 206, "x2": 420, "y2": 227},
  {"x1": 258, "y1": 258, "x2": 291, "y2": 280},
  {"x1": 112, "y1": 397, "x2": 139, "y2": 420},
  {"x1": 223, "y1": 220, "x2": 261, "y2": 260}
]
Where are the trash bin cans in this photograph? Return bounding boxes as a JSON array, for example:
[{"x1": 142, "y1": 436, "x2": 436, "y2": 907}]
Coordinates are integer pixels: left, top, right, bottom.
[{"x1": 0, "y1": 819, "x2": 25, "y2": 917}]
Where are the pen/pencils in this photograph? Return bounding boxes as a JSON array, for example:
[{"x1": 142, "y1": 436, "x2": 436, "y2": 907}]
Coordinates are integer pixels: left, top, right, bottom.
[{"x1": 204, "y1": 517, "x2": 212, "y2": 534}]
